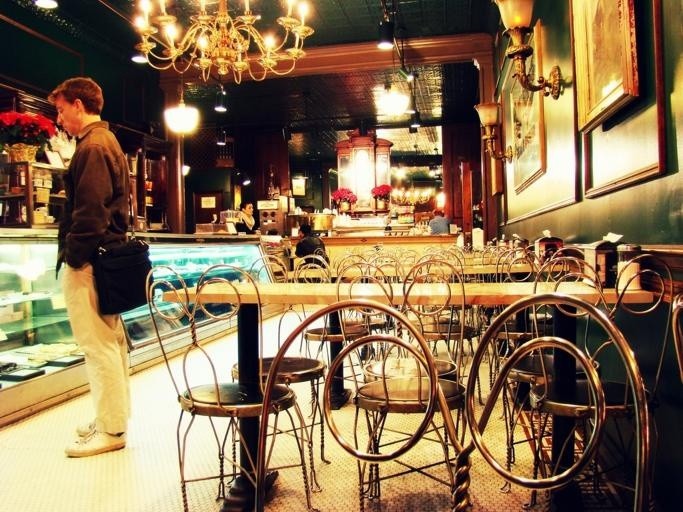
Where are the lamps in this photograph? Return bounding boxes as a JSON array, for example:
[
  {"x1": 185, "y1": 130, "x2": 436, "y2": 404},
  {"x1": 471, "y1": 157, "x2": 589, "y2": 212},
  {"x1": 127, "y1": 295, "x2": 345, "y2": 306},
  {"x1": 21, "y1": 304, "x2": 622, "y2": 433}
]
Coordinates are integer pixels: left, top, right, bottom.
[
  {"x1": 375, "y1": 1, "x2": 422, "y2": 136},
  {"x1": 124, "y1": 0, "x2": 318, "y2": 88},
  {"x1": 491, "y1": 1, "x2": 564, "y2": 102},
  {"x1": 472, "y1": 99, "x2": 514, "y2": 165}
]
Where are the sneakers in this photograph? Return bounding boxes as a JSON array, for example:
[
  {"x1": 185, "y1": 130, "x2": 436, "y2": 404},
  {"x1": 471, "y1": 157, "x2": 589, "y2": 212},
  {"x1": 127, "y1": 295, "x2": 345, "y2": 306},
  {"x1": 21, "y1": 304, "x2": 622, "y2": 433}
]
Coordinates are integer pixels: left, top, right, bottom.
[
  {"x1": 76, "y1": 419, "x2": 97, "y2": 438},
  {"x1": 65, "y1": 428, "x2": 126, "y2": 458}
]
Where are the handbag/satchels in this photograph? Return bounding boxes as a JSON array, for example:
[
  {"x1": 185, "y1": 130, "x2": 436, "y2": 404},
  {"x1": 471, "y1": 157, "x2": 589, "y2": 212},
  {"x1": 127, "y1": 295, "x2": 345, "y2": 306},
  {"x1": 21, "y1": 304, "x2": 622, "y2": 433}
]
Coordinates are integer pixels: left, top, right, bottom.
[{"x1": 92, "y1": 238, "x2": 155, "y2": 315}]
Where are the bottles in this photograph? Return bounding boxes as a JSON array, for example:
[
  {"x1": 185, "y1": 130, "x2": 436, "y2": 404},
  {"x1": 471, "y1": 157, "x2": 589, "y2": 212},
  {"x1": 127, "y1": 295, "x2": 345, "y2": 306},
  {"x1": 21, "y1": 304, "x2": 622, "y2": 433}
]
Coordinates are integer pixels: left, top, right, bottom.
[{"x1": 614, "y1": 243, "x2": 641, "y2": 291}]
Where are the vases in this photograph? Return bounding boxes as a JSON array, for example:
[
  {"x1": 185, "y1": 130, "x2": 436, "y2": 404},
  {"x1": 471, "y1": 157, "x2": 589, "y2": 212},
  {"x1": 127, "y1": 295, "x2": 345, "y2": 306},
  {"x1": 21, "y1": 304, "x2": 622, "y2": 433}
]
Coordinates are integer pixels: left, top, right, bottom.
[
  {"x1": 2, "y1": 143, "x2": 41, "y2": 163},
  {"x1": 377, "y1": 198, "x2": 387, "y2": 210},
  {"x1": 338, "y1": 201, "x2": 351, "y2": 211}
]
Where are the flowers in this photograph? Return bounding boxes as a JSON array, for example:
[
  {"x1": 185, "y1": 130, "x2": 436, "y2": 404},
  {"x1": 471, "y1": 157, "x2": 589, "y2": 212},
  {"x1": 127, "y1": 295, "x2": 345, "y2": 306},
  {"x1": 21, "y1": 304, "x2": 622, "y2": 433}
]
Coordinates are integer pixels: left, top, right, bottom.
[
  {"x1": 369, "y1": 184, "x2": 392, "y2": 198},
  {"x1": 330, "y1": 188, "x2": 356, "y2": 204},
  {"x1": 0, "y1": 111, "x2": 57, "y2": 145}
]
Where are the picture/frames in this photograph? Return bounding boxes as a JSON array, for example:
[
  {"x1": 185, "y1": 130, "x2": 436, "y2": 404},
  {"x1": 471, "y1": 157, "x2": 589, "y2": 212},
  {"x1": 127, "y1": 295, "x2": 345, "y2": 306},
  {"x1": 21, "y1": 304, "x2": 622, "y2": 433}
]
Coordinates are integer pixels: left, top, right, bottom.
[
  {"x1": 566, "y1": 0, "x2": 642, "y2": 137},
  {"x1": 506, "y1": 19, "x2": 548, "y2": 196}
]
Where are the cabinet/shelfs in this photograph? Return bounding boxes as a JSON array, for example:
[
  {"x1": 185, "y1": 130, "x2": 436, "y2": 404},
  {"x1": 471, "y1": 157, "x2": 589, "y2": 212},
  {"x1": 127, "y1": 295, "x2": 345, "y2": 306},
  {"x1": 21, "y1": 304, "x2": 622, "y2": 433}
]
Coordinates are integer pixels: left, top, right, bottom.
[
  {"x1": 0, "y1": 226, "x2": 275, "y2": 430},
  {"x1": 0, "y1": 161, "x2": 64, "y2": 228}
]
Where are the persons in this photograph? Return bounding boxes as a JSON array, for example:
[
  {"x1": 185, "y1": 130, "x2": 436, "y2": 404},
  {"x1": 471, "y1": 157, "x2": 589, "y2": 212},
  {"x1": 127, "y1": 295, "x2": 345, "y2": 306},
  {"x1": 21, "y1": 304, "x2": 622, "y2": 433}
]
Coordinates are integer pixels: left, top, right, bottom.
[
  {"x1": 237, "y1": 202, "x2": 260, "y2": 233},
  {"x1": 296, "y1": 224, "x2": 329, "y2": 284},
  {"x1": 429, "y1": 209, "x2": 451, "y2": 234},
  {"x1": 45, "y1": 76, "x2": 130, "y2": 458}
]
